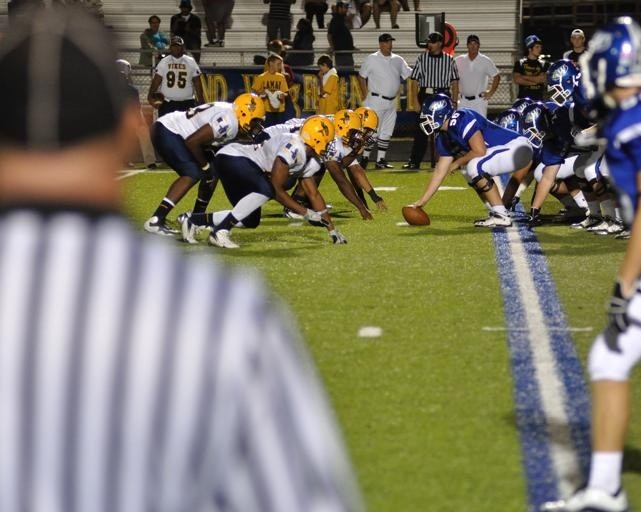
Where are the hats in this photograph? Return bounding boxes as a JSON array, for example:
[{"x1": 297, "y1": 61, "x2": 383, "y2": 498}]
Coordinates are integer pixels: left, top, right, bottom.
[
  {"x1": 378, "y1": 33, "x2": 396, "y2": 41},
  {"x1": 466, "y1": 34, "x2": 481, "y2": 45},
  {"x1": 169, "y1": 35, "x2": 185, "y2": 48},
  {"x1": 335, "y1": 1, "x2": 349, "y2": 7},
  {"x1": 424, "y1": 31, "x2": 446, "y2": 43},
  {"x1": 0, "y1": 11, "x2": 129, "y2": 147},
  {"x1": 524, "y1": 35, "x2": 542, "y2": 46},
  {"x1": 572, "y1": 28, "x2": 585, "y2": 38},
  {"x1": 178, "y1": 0, "x2": 193, "y2": 10},
  {"x1": 115, "y1": 58, "x2": 132, "y2": 77}
]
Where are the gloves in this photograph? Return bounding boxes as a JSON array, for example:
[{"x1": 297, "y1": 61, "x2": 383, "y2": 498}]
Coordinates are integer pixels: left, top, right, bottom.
[
  {"x1": 526, "y1": 207, "x2": 543, "y2": 231},
  {"x1": 607, "y1": 279, "x2": 634, "y2": 333},
  {"x1": 328, "y1": 229, "x2": 349, "y2": 245},
  {"x1": 304, "y1": 208, "x2": 331, "y2": 229}
]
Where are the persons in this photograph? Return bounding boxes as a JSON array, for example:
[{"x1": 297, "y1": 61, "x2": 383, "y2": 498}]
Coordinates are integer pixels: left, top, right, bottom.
[
  {"x1": 136, "y1": 0, "x2": 641, "y2": 259},
  {"x1": 113, "y1": 57, "x2": 159, "y2": 171},
  {"x1": 2, "y1": 5, "x2": 370, "y2": 511},
  {"x1": 539, "y1": 14, "x2": 641, "y2": 512}
]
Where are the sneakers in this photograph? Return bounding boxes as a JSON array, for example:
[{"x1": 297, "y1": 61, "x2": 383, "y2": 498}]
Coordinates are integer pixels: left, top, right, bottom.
[
  {"x1": 473, "y1": 208, "x2": 513, "y2": 228},
  {"x1": 551, "y1": 206, "x2": 632, "y2": 240},
  {"x1": 206, "y1": 227, "x2": 240, "y2": 250},
  {"x1": 143, "y1": 214, "x2": 176, "y2": 238},
  {"x1": 177, "y1": 209, "x2": 204, "y2": 245},
  {"x1": 358, "y1": 156, "x2": 421, "y2": 171},
  {"x1": 539, "y1": 484, "x2": 630, "y2": 512},
  {"x1": 284, "y1": 195, "x2": 334, "y2": 220}
]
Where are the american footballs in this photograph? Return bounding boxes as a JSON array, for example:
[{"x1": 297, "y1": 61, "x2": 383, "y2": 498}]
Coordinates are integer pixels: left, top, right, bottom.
[
  {"x1": 402, "y1": 205, "x2": 430, "y2": 226},
  {"x1": 153, "y1": 91, "x2": 164, "y2": 109}
]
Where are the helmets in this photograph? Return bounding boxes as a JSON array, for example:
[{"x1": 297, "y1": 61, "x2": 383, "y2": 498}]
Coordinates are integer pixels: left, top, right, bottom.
[
  {"x1": 300, "y1": 115, "x2": 337, "y2": 167},
  {"x1": 354, "y1": 106, "x2": 380, "y2": 152},
  {"x1": 232, "y1": 93, "x2": 266, "y2": 140},
  {"x1": 332, "y1": 109, "x2": 364, "y2": 149},
  {"x1": 578, "y1": 14, "x2": 641, "y2": 100},
  {"x1": 418, "y1": 93, "x2": 454, "y2": 136},
  {"x1": 492, "y1": 97, "x2": 552, "y2": 149},
  {"x1": 547, "y1": 59, "x2": 581, "y2": 109}
]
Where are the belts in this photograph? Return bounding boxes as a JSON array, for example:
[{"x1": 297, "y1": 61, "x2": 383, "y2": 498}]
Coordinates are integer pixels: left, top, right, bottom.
[
  {"x1": 372, "y1": 92, "x2": 397, "y2": 101},
  {"x1": 460, "y1": 94, "x2": 481, "y2": 101}
]
